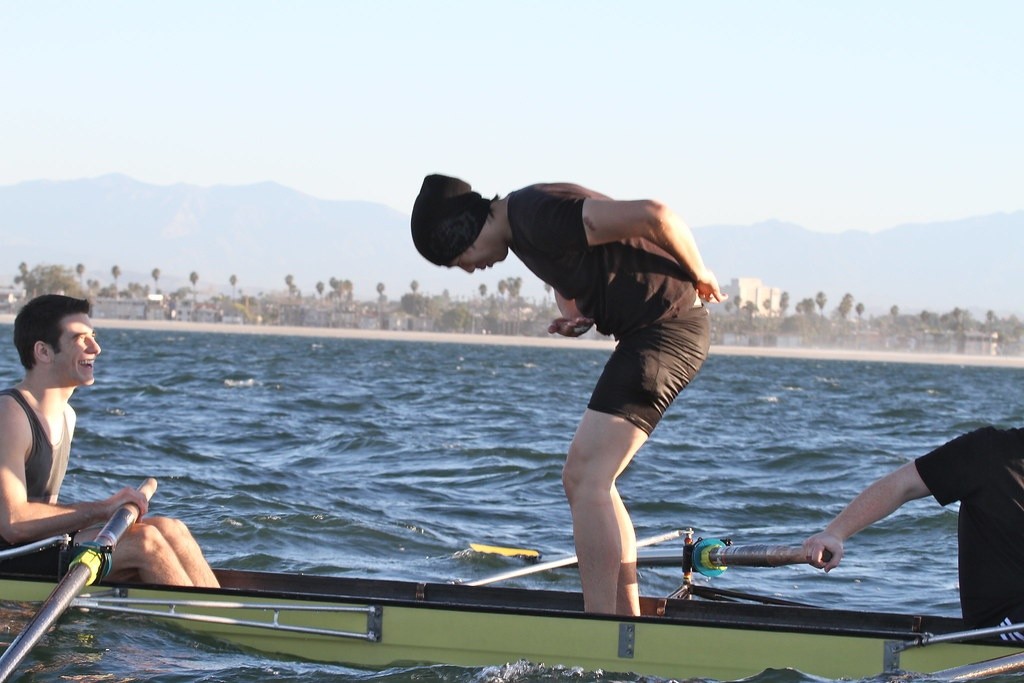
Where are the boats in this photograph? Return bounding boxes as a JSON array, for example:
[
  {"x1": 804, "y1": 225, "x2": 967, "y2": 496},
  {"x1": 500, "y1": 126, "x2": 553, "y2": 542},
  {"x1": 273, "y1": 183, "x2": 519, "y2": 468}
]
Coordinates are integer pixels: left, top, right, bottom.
[{"x1": 1, "y1": 564, "x2": 1024, "y2": 683}]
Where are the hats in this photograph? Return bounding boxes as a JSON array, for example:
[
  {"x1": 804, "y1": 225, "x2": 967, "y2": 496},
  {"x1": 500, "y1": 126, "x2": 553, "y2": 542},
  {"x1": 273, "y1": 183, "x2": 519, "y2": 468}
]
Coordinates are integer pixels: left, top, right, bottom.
[{"x1": 411, "y1": 174, "x2": 488, "y2": 265}]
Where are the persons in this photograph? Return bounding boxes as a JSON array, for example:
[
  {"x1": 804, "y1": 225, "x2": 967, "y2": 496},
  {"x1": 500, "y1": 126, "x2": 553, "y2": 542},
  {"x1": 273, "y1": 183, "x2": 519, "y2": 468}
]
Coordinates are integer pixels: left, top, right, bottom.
[
  {"x1": 0, "y1": 294, "x2": 220, "y2": 588},
  {"x1": 802, "y1": 426, "x2": 1024, "y2": 640},
  {"x1": 412, "y1": 174, "x2": 728, "y2": 616}
]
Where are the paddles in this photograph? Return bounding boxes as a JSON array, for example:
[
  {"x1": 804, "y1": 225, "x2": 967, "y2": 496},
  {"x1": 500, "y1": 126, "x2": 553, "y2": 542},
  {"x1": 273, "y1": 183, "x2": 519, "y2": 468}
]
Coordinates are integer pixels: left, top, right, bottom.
[
  {"x1": 0, "y1": 477, "x2": 159, "y2": 683},
  {"x1": 470, "y1": 535, "x2": 839, "y2": 577}
]
